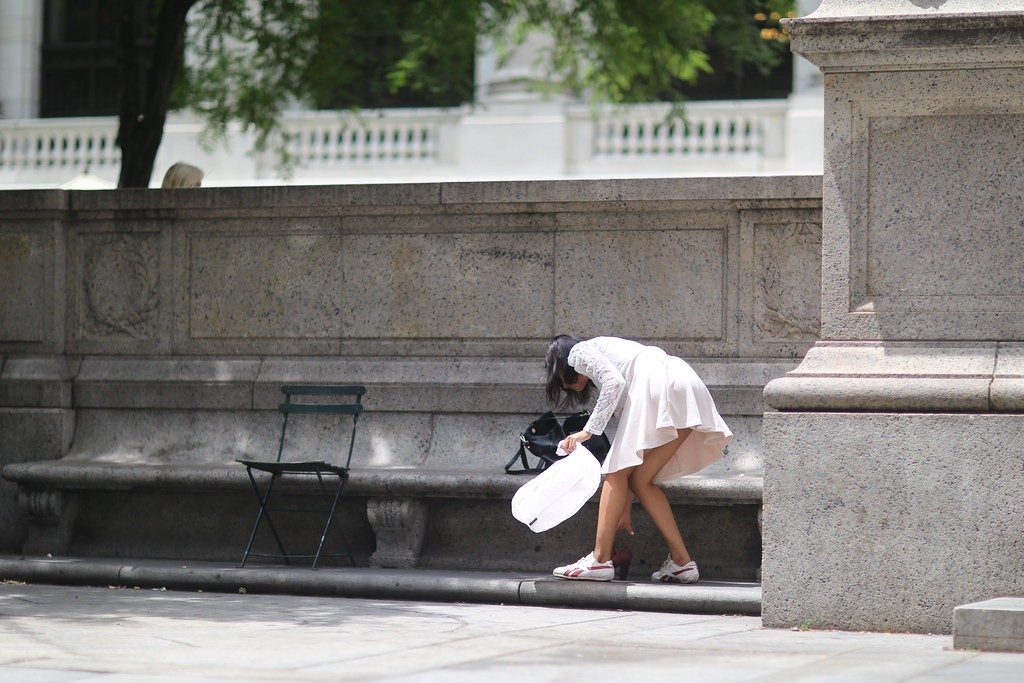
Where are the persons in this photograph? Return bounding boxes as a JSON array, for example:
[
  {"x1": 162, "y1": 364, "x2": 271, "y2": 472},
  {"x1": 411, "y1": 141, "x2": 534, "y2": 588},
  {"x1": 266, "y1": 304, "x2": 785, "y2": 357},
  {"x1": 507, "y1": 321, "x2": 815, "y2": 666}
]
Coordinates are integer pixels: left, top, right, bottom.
[{"x1": 545, "y1": 335, "x2": 734, "y2": 584}]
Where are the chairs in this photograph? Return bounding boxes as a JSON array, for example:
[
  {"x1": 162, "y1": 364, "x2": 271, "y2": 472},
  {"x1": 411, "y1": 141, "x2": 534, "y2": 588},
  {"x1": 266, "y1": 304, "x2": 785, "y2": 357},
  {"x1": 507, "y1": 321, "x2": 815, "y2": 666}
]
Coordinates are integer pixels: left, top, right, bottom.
[{"x1": 234, "y1": 385, "x2": 369, "y2": 569}]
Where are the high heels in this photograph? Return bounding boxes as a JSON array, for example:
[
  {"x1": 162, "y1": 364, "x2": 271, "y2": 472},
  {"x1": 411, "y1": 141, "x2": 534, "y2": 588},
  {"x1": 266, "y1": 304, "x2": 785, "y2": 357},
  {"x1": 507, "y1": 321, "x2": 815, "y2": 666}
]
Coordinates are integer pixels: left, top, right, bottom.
[{"x1": 611, "y1": 546, "x2": 631, "y2": 581}]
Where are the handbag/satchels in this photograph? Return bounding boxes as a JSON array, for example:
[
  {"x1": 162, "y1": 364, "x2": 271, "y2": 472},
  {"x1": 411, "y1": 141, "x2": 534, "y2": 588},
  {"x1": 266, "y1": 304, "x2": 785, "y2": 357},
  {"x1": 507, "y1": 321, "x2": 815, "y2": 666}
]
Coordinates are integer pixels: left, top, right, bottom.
[
  {"x1": 511, "y1": 439, "x2": 602, "y2": 533},
  {"x1": 503, "y1": 411, "x2": 611, "y2": 474}
]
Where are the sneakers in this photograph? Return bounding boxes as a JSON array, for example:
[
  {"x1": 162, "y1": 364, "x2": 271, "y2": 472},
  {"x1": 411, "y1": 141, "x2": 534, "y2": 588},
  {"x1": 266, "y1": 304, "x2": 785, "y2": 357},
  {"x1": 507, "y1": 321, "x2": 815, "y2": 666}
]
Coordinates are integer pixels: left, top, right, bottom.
[
  {"x1": 650, "y1": 553, "x2": 699, "y2": 583},
  {"x1": 553, "y1": 551, "x2": 615, "y2": 581}
]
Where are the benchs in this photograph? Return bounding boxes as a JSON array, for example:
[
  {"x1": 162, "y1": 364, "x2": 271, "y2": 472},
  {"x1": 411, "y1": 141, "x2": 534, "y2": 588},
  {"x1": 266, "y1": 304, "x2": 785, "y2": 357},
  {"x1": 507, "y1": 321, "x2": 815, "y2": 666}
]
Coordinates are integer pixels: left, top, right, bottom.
[{"x1": 0, "y1": 408, "x2": 765, "y2": 570}]
